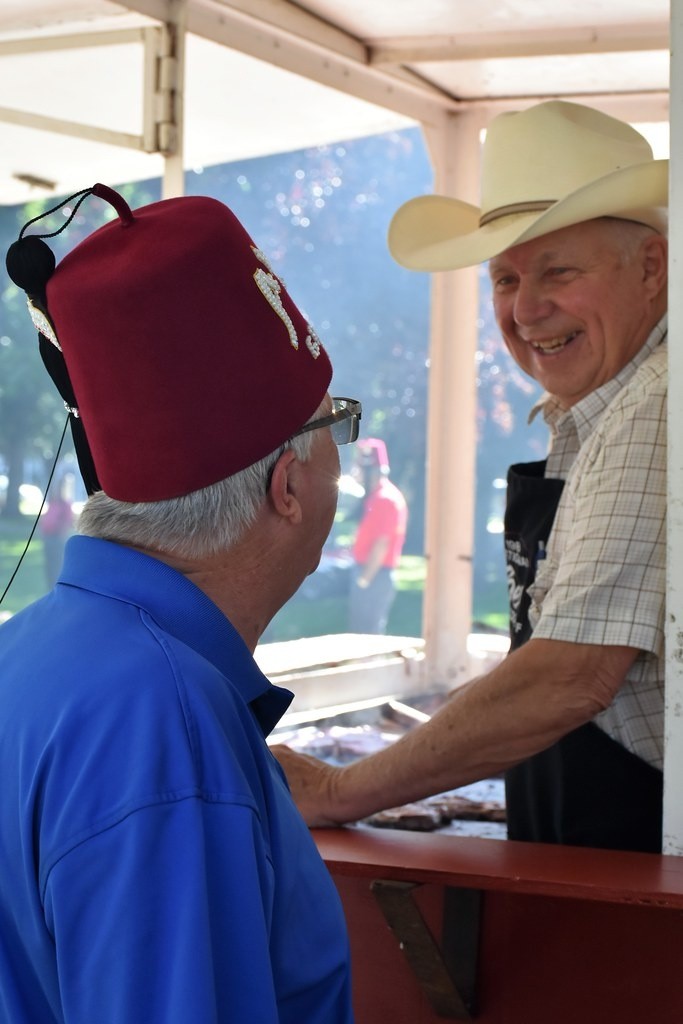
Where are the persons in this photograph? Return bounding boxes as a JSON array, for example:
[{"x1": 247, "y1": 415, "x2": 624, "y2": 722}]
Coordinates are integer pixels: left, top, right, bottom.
[
  {"x1": 38, "y1": 480, "x2": 73, "y2": 589},
  {"x1": 265, "y1": 102, "x2": 669, "y2": 851},
  {"x1": 349, "y1": 437, "x2": 408, "y2": 634},
  {"x1": 0, "y1": 181, "x2": 363, "y2": 1024}
]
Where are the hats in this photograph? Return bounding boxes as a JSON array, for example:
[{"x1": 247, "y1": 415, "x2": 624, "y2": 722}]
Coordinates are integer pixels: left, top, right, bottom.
[
  {"x1": 384, "y1": 98, "x2": 671, "y2": 281},
  {"x1": 351, "y1": 436, "x2": 390, "y2": 472},
  {"x1": 4, "y1": 181, "x2": 336, "y2": 503}
]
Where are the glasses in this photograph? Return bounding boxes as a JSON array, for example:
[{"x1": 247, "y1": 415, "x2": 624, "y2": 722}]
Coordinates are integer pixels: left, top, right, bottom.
[{"x1": 282, "y1": 394, "x2": 365, "y2": 448}]
